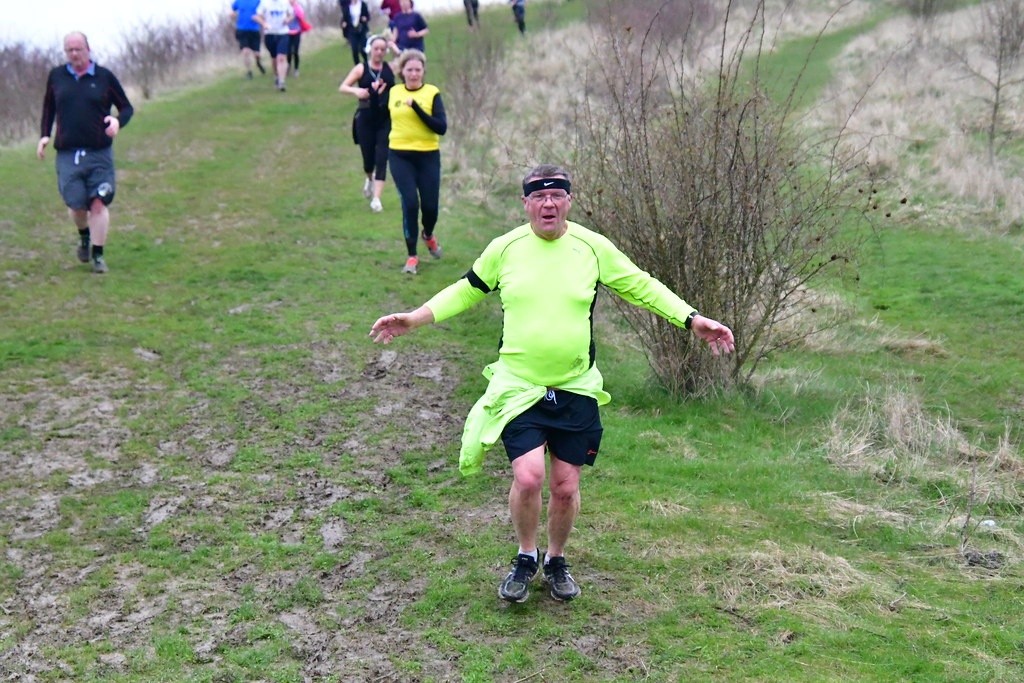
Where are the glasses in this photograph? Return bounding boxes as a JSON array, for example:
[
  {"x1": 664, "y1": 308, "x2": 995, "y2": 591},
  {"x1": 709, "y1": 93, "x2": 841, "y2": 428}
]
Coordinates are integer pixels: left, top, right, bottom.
[{"x1": 527, "y1": 193, "x2": 568, "y2": 202}]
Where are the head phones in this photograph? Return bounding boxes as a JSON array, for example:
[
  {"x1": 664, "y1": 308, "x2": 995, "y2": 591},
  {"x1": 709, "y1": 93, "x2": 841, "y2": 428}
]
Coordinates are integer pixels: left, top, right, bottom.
[{"x1": 365, "y1": 35, "x2": 389, "y2": 54}]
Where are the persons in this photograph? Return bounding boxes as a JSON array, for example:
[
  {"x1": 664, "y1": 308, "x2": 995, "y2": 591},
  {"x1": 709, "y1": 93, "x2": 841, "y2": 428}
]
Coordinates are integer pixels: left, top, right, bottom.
[
  {"x1": 231, "y1": 0, "x2": 313, "y2": 91},
  {"x1": 464, "y1": 0, "x2": 480, "y2": 32},
  {"x1": 368, "y1": 49, "x2": 447, "y2": 274},
  {"x1": 339, "y1": 0, "x2": 429, "y2": 65},
  {"x1": 369, "y1": 165, "x2": 735, "y2": 602},
  {"x1": 37, "y1": 32, "x2": 133, "y2": 273},
  {"x1": 511, "y1": 0, "x2": 527, "y2": 35},
  {"x1": 339, "y1": 32, "x2": 395, "y2": 212}
]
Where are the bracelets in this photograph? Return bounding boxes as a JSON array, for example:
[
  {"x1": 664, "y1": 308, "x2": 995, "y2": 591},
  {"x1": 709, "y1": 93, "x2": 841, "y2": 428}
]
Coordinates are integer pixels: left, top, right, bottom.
[{"x1": 685, "y1": 312, "x2": 698, "y2": 330}]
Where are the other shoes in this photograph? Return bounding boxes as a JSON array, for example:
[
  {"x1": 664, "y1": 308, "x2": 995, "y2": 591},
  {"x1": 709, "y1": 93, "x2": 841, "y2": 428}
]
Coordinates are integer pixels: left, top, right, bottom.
[
  {"x1": 278, "y1": 79, "x2": 286, "y2": 92},
  {"x1": 274, "y1": 73, "x2": 279, "y2": 84}
]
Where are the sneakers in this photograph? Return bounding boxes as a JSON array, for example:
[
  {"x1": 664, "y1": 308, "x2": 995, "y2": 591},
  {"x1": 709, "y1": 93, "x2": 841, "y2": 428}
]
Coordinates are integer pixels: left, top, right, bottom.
[
  {"x1": 247, "y1": 71, "x2": 253, "y2": 80},
  {"x1": 402, "y1": 256, "x2": 419, "y2": 274},
  {"x1": 94, "y1": 259, "x2": 109, "y2": 273},
  {"x1": 542, "y1": 551, "x2": 581, "y2": 601},
  {"x1": 370, "y1": 198, "x2": 383, "y2": 212},
  {"x1": 78, "y1": 239, "x2": 91, "y2": 262},
  {"x1": 422, "y1": 229, "x2": 442, "y2": 259},
  {"x1": 498, "y1": 548, "x2": 540, "y2": 602},
  {"x1": 364, "y1": 178, "x2": 372, "y2": 198},
  {"x1": 257, "y1": 65, "x2": 266, "y2": 73}
]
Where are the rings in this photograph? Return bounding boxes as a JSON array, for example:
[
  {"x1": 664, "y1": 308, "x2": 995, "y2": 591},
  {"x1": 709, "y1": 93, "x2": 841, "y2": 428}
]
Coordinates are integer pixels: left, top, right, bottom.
[{"x1": 717, "y1": 338, "x2": 720, "y2": 340}]
